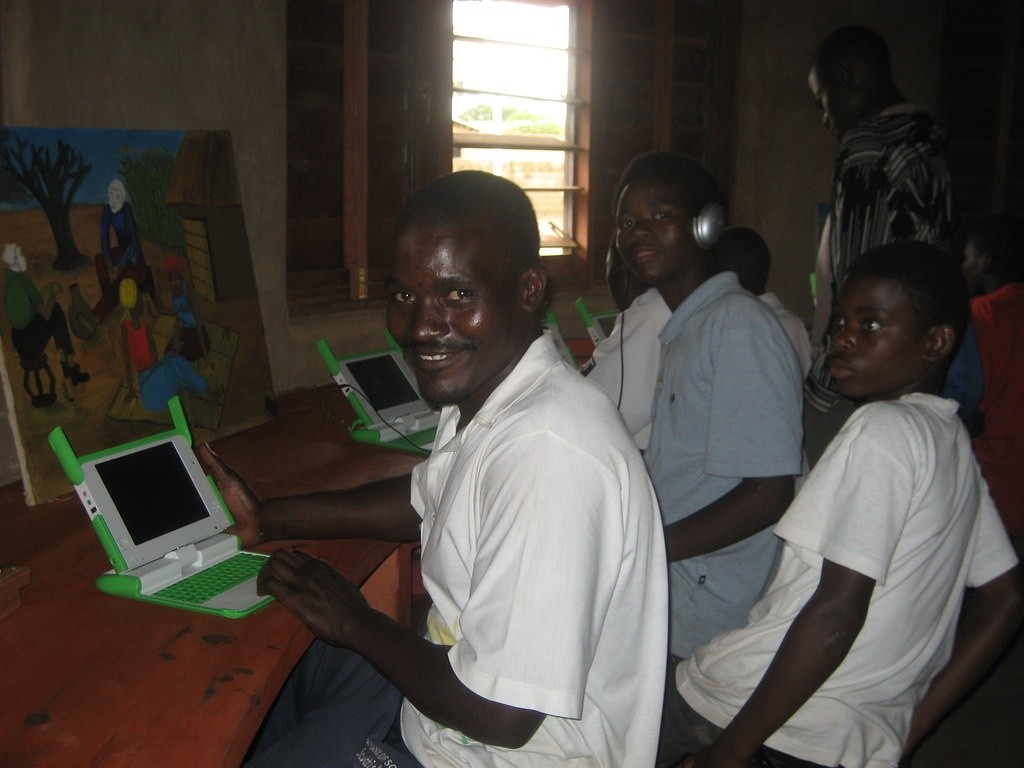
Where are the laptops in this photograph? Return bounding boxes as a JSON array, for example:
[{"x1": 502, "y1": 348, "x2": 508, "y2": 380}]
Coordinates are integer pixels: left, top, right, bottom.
[
  {"x1": 315, "y1": 330, "x2": 443, "y2": 457},
  {"x1": 576, "y1": 297, "x2": 620, "y2": 350},
  {"x1": 808, "y1": 272, "x2": 820, "y2": 305},
  {"x1": 45, "y1": 395, "x2": 276, "y2": 618},
  {"x1": 540, "y1": 309, "x2": 581, "y2": 376}
]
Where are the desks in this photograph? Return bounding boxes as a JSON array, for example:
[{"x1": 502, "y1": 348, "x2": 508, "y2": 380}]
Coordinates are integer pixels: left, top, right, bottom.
[{"x1": 0, "y1": 378, "x2": 427, "y2": 768}]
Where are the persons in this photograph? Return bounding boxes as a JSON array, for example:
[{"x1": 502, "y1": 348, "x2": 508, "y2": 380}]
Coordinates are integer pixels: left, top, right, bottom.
[
  {"x1": 200, "y1": 170, "x2": 670, "y2": 768},
  {"x1": 657, "y1": 238, "x2": 1024, "y2": 768},
  {"x1": 580, "y1": 22, "x2": 1023, "y2": 696}
]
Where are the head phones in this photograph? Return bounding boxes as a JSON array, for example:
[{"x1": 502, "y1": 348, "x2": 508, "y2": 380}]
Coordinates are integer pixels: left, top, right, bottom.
[{"x1": 690, "y1": 200, "x2": 726, "y2": 251}]
